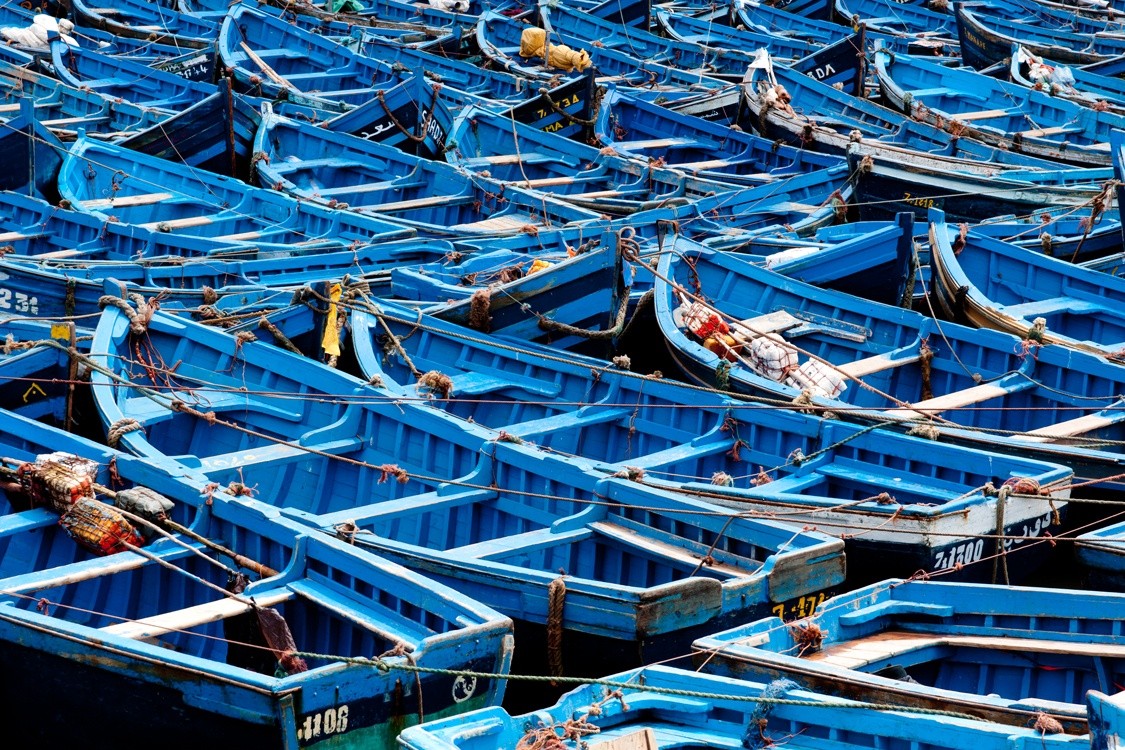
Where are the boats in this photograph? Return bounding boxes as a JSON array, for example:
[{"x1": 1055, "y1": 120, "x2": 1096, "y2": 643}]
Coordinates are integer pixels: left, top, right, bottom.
[{"x1": 0, "y1": 0, "x2": 1125, "y2": 750}]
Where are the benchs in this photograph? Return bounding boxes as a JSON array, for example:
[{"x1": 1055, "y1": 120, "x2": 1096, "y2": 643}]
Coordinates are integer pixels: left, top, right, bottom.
[{"x1": 0, "y1": 10, "x2": 1125, "y2": 673}]
